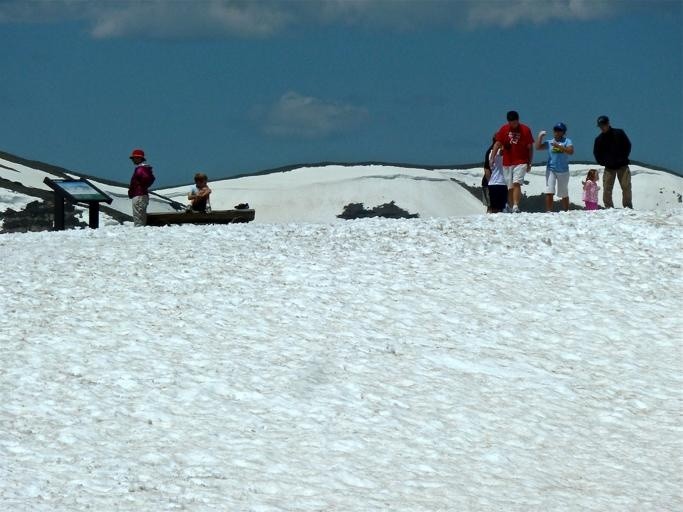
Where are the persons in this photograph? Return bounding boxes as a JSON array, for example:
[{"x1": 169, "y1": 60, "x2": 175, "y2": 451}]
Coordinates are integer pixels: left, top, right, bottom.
[
  {"x1": 128, "y1": 149, "x2": 155, "y2": 226},
  {"x1": 536, "y1": 122, "x2": 573, "y2": 212},
  {"x1": 481, "y1": 111, "x2": 535, "y2": 212},
  {"x1": 593, "y1": 116, "x2": 632, "y2": 208},
  {"x1": 188, "y1": 174, "x2": 210, "y2": 212},
  {"x1": 582, "y1": 169, "x2": 600, "y2": 210}
]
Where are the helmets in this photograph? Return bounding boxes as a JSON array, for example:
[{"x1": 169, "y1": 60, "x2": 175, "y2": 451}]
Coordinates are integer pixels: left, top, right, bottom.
[
  {"x1": 553, "y1": 121, "x2": 567, "y2": 131},
  {"x1": 596, "y1": 116, "x2": 609, "y2": 127}
]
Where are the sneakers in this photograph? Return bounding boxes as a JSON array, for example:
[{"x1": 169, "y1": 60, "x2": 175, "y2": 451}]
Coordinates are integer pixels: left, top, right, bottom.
[{"x1": 503, "y1": 203, "x2": 521, "y2": 213}]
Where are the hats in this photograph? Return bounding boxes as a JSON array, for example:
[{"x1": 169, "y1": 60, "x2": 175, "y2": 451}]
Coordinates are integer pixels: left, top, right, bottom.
[{"x1": 130, "y1": 150, "x2": 146, "y2": 161}]
